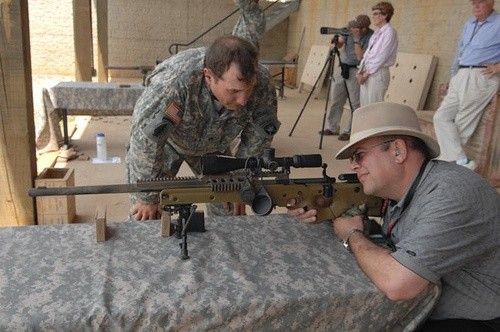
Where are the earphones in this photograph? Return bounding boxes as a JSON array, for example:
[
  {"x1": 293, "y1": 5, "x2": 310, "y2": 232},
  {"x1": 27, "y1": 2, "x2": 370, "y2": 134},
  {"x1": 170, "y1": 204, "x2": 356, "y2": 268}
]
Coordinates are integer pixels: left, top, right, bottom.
[{"x1": 396, "y1": 149, "x2": 401, "y2": 156}]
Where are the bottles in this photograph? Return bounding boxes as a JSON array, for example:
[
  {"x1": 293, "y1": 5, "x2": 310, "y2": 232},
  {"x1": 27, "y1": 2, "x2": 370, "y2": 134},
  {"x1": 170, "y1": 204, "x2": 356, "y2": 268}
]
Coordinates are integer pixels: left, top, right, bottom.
[{"x1": 96, "y1": 134, "x2": 106, "y2": 160}]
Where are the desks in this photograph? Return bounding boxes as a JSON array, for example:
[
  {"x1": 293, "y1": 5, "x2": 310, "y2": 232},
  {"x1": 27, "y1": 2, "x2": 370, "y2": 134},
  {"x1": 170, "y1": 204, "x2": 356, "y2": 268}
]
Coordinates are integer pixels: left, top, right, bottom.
[
  {"x1": 0, "y1": 207, "x2": 442, "y2": 332},
  {"x1": 35, "y1": 81, "x2": 145, "y2": 157},
  {"x1": 260, "y1": 60, "x2": 298, "y2": 101}
]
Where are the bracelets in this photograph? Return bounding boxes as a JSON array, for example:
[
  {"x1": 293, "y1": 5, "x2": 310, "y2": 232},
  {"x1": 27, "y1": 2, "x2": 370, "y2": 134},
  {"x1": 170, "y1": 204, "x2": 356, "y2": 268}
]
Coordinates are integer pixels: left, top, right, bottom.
[{"x1": 354, "y1": 40, "x2": 362, "y2": 46}]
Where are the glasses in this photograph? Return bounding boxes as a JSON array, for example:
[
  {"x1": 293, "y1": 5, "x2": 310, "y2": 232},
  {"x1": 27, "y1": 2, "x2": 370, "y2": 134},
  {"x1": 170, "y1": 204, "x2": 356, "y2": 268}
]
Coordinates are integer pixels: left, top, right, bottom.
[
  {"x1": 349, "y1": 140, "x2": 394, "y2": 164},
  {"x1": 372, "y1": 12, "x2": 381, "y2": 16}
]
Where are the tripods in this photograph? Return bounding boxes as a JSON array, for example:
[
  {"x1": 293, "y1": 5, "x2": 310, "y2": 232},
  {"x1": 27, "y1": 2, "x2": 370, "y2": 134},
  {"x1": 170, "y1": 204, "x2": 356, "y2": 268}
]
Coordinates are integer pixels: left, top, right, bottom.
[{"x1": 289, "y1": 35, "x2": 353, "y2": 150}]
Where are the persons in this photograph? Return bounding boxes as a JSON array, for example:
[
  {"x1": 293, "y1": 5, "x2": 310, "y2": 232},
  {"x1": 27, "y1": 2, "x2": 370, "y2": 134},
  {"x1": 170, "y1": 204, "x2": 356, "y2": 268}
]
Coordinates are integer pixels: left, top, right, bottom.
[
  {"x1": 432, "y1": 0, "x2": 500, "y2": 172},
  {"x1": 319, "y1": 15, "x2": 375, "y2": 141},
  {"x1": 231, "y1": 0, "x2": 267, "y2": 50},
  {"x1": 124, "y1": 33, "x2": 282, "y2": 221},
  {"x1": 356, "y1": 1, "x2": 398, "y2": 107},
  {"x1": 286, "y1": 102, "x2": 500, "y2": 332}
]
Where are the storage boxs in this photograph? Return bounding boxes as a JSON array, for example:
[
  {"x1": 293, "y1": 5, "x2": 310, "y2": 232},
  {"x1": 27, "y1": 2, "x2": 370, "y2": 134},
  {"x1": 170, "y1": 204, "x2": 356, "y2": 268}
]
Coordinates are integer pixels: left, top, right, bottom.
[{"x1": 35, "y1": 167, "x2": 76, "y2": 225}]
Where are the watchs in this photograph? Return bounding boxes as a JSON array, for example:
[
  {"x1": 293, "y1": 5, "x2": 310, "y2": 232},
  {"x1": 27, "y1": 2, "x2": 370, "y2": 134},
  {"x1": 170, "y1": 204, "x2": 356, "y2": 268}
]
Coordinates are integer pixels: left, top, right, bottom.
[{"x1": 341, "y1": 228, "x2": 366, "y2": 253}]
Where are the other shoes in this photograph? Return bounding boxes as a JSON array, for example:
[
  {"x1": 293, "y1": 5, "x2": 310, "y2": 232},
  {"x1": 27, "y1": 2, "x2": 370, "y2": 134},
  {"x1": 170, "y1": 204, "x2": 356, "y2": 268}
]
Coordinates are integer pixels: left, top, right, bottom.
[
  {"x1": 338, "y1": 134, "x2": 349, "y2": 140},
  {"x1": 460, "y1": 159, "x2": 477, "y2": 171},
  {"x1": 319, "y1": 129, "x2": 339, "y2": 135}
]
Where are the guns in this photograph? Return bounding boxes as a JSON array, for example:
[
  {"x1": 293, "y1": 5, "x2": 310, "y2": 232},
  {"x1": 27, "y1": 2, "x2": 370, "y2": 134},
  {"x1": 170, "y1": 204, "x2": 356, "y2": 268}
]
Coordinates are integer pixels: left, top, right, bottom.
[
  {"x1": 27, "y1": 147, "x2": 387, "y2": 261},
  {"x1": 105, "y1": 60, "x2": 163, "y2": 86}
]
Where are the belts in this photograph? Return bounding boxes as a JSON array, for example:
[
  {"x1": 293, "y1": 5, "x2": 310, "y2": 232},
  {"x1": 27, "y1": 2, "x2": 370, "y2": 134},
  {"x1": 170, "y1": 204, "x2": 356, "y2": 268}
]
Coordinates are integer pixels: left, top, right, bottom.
[{"x1": 459, "y1": 66, "x2": 488, "y2": 68}]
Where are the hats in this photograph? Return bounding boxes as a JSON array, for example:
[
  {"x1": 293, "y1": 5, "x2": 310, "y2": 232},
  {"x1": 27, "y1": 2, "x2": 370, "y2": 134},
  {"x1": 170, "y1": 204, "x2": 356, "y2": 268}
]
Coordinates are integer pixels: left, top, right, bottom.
[
  {"x1": 335, "y1": 102, "x2": 440, "y2": 159},
  {"x1": 348, "y1": 15, "x2": 371, "y2": 28}
]
